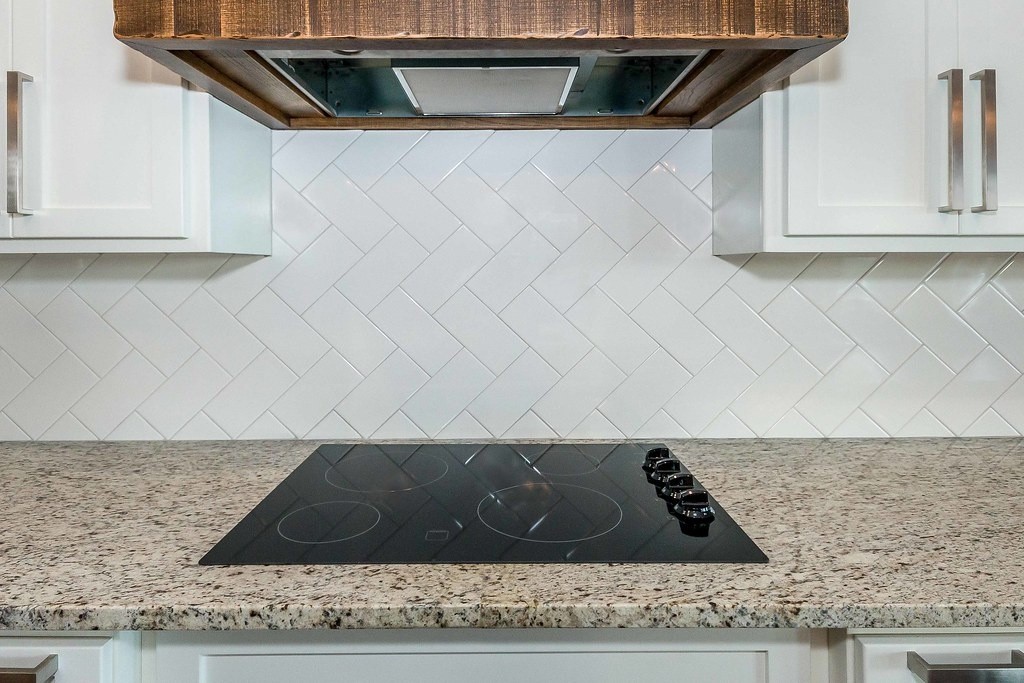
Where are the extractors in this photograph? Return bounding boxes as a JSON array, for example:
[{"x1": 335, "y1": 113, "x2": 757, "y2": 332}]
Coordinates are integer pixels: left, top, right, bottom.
[{"x1": 254, "y1": 47, "x2": 713, "y2": 118}]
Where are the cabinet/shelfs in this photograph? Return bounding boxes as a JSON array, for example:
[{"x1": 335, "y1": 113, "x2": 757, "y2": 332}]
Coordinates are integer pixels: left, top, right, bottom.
[
  {"x1": 828, "y1": 627, "x2": 1024, "y2": 683},
  {"x1": 0, "y1": 0, "x2": 272, "y2": 256},
  {"x1": 154, "y1": 628, "x2": 811, "y2": 683},
  {"x1": 0, "y1": 630, "x2": 142, "y2": 683},
  {"x1": 711, "y1": 0, "x2": 1024, "y2": 256}
]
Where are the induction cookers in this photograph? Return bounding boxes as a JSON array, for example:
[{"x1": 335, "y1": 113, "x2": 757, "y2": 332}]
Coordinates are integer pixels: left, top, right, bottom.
[{"x1": 198, "y1": 443, "x2": 768, "y2": 569}]
